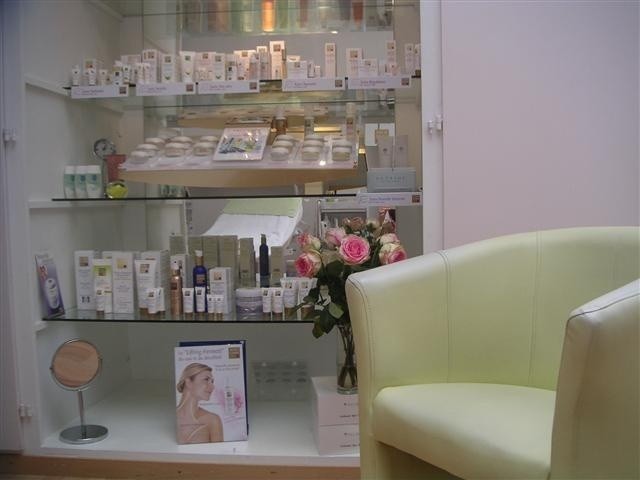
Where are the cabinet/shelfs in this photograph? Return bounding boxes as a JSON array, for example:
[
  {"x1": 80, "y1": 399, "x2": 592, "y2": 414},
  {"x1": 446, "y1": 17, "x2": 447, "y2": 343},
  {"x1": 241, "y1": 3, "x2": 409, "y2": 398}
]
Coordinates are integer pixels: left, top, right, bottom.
[{"x1": 1, "y1": 0, "x2": 445, "y2": 467}]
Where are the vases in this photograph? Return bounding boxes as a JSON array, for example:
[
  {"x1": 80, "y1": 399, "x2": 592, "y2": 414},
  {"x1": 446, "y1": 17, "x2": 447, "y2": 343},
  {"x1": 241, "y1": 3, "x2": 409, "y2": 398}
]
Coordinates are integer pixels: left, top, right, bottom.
[{"x1": 336, "y1": 324, "x2": 356, "y2": 395}]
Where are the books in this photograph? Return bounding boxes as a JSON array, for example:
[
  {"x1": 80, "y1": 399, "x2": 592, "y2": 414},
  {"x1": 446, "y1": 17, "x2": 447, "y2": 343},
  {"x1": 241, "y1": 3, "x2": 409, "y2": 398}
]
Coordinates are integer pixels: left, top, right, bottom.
[
  {"x1": 171, "y1": 344, "x2": 249, "y2": 448},
  {"x1": 180, "y1": 338, "x2": 249, "y2": 437}
]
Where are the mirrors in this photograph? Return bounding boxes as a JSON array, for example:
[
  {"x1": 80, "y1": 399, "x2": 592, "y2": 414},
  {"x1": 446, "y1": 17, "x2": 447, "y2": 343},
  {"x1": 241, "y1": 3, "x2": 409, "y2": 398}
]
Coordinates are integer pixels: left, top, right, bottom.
[{"x1": 49, "y1": 338, "x2": 108, "y2": 445}]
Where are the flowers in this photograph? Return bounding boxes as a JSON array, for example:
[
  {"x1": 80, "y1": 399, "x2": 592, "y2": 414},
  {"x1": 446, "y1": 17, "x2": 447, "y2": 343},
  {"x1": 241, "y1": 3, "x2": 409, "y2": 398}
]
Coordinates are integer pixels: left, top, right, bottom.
[{"x1": 288, "y1": 217, "x2": 407, "y2": 388}]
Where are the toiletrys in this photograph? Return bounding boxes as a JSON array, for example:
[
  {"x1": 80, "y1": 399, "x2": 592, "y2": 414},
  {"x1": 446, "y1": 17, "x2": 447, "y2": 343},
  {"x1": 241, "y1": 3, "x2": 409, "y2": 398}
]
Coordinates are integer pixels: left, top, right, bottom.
[
  {"x1": 72, "y1": 233, "x2": 317, "y2": 320},
  {"x1": 63, "y1": 38, "x2": 422, "y2": 92},
  {"x1": 63, "y1": 102, "x2": 418, "y2": 198}
]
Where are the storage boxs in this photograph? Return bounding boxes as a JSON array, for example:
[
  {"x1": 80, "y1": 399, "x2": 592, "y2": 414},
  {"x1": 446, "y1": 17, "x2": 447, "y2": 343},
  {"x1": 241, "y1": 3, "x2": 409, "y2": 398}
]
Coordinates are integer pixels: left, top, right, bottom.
[
  {"x1": 309, "y1": 376, "x2": 359, "y2": 426},
  {"x1": 311, "y1": 407, "x2": 360, "y2": 456}
]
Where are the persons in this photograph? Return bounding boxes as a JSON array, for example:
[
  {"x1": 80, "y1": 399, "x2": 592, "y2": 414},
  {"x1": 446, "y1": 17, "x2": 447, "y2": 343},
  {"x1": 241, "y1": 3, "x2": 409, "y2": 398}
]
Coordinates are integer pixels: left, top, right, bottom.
[{"x1": 175, "y1": 362, "x2": 225, "y2": 445}]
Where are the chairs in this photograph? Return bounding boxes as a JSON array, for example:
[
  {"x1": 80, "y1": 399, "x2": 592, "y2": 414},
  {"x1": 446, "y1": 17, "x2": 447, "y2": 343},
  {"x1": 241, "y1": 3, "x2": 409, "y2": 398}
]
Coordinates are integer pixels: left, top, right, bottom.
[{"x1": 344, "y1": 225, "x2": 640, "y2": 480}]
